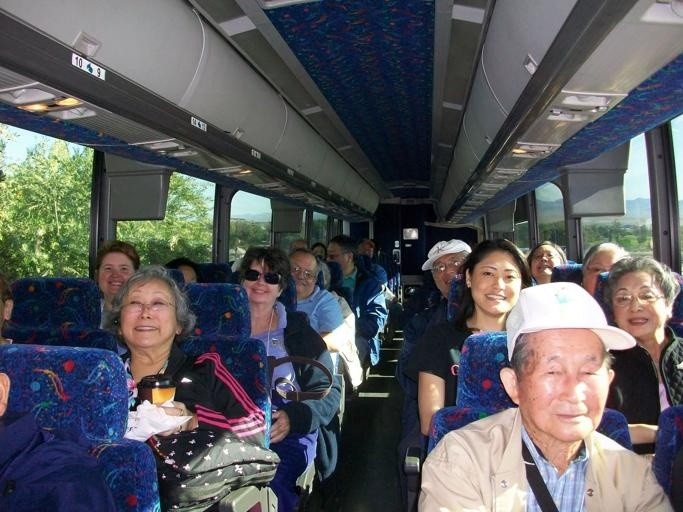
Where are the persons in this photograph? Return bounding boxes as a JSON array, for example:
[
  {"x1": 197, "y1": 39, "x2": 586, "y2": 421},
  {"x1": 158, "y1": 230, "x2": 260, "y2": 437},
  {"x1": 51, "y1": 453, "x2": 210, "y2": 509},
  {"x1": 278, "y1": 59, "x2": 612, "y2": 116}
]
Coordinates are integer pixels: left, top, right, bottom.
[
  {"x1": 526, "y1": 240, "x2": 568, "y2": 287},
  {"x1": 601, "y1": 256, "x2": 683, "y2": 455},
  {"x1": 400, "y1": 237, "x2": 533, "y2": 438},
  {"x1": 0, "y1": 235, "x2": 398, "y2": 512},
  {"x1": 579, "y1": 242, "x2": 633, "y2": 297},
  {"x1": 414, "y1": 279, "x2": 678, "y2": 511},
  {"x1": 396, "y1": 235, "x2": 474, "y2": 392}
]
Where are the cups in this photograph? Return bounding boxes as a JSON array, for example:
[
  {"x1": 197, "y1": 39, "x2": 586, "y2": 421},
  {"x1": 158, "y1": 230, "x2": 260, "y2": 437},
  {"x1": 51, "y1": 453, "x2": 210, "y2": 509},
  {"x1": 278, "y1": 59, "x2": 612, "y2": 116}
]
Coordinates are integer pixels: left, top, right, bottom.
[{"x1": 134, "y1": 373, "x2": 176, "y2": 404}]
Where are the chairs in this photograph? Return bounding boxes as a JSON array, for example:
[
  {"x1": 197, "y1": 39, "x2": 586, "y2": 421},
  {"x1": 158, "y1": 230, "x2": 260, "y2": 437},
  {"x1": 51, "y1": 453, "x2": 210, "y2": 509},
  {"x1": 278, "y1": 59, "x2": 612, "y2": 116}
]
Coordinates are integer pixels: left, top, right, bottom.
[
  {"x1": 404, "y1": 261, "x2": 681, "y2": 510},
  {"x1": 1, "y1": 253, "x2": 402, "y2": 512}
]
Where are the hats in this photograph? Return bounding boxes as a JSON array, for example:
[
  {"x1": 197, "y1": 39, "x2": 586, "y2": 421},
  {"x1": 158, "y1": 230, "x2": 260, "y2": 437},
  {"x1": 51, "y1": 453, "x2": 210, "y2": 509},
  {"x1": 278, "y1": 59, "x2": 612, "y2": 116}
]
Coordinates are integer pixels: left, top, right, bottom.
[
  {"x1": 421, "y1": 238, "x2": 472, "y2": 271},
  {"x1": 508, "y1": 281, "x2": 636, "y2": 361}
]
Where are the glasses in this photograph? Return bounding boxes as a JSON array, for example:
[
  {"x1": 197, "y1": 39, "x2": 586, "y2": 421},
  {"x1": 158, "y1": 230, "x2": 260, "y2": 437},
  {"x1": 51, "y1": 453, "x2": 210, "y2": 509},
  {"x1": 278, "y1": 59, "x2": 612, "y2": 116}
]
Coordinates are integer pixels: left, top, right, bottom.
[
  {"x1": 329, "y1": 254, "x2": 340, "y2": 260},
  {"x1": 434, "y1": 258, "x2": 463, "y2": 272},
  {"x1": 613, "y1": 292, "x2": 665, "y2": 306},
  {"x1": 130, "y1": 297, "x2": 175, "y2": 312},
  {"x1": 291, "y1": 267, "x2": 316, "y2": 280},
  {"x1": 244, "y1": 270, "x2": 279, "y2": 284}
]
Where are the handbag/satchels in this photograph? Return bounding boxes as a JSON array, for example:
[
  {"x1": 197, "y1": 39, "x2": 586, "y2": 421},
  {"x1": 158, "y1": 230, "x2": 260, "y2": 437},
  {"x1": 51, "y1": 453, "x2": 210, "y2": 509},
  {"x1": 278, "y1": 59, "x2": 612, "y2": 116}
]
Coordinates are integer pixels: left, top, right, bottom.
[
  {"x1": 148, "y1": 428, "x2": 279, "y2": 512},
  {"x1": 266, "y1": 357, "x2": 333, "y2": 401}
]
